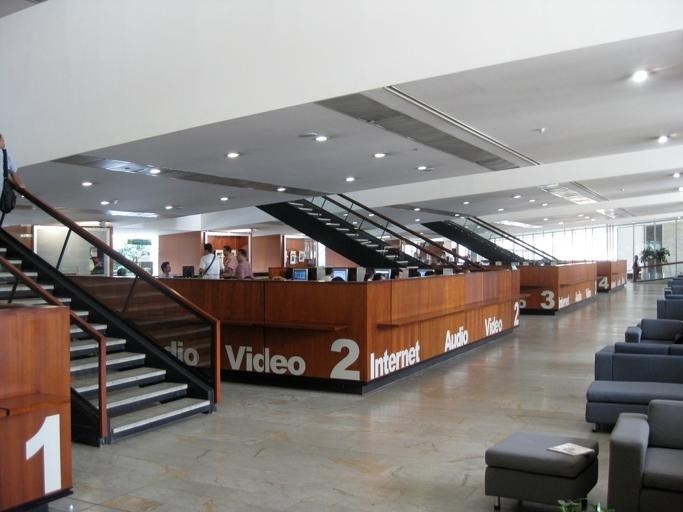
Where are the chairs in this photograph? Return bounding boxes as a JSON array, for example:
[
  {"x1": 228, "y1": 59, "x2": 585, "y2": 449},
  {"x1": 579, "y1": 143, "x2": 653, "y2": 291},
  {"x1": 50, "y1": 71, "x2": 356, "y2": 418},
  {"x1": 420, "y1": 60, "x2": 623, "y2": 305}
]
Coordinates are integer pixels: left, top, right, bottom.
[{"x1": 607, "y1": 398, "x2": 683, "y2": 512}]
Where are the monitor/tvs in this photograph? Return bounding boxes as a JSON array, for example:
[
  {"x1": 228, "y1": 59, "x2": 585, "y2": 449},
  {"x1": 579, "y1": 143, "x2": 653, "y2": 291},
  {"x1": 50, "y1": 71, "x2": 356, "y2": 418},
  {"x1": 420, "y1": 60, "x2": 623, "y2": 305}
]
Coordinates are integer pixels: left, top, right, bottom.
[
  {"x1": 416, "y1": 269, "x2": 435, "y2": 277},
  {"x1": 331, "y1": 268, "x2": 349, "y2": 281},
  {"x1": 374, "y1": 268, "x2": 391, "y2": 280},
  {"x1": 183, "y1": 266, "x2": 194, "y2": 278},
  {"x1": 292, "y1": 268, "x2": 309, "y2": 280}
]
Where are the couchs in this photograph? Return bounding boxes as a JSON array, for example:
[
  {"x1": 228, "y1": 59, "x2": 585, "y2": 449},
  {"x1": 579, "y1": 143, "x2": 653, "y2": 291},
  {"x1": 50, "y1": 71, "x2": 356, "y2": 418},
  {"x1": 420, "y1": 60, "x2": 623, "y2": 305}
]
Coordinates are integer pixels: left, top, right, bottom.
[{"x1": 584, "y1": 273, "x2": 683, "y2": 430}]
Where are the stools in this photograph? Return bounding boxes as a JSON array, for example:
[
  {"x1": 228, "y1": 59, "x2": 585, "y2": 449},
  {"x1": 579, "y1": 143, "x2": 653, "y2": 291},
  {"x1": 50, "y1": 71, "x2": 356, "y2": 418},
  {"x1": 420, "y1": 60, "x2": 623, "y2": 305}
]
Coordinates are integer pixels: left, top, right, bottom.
[{"x1": 484, "y1": 431, "x2": 599, "y2": 511}]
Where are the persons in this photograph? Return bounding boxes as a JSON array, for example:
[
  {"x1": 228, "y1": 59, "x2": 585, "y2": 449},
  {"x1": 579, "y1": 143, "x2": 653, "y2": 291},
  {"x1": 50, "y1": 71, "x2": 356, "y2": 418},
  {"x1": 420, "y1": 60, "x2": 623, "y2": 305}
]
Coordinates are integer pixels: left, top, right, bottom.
[
  {"x1": 198, "y1": 243, "x2": 223, "y2": 280},
  {"x1": 221, "y1": 244, "x2": 238, "y2": 278},
  {"x1": 235, "y1": 249, "x2": 254, "y2": 279},
  {"x1": 0, "y1": 134, "x2": 25, "y2": 221},
  {"x1": 632, "y1": 255, "x2": 640, "y2": 282},
  {"x1": 372, "y1": 273, "x2": 386, "y2": 281},
  {"x1": 363, "y1": 272, "x2": 374, "y2": 282},
  {"x1": 158, "y1": 261, "x2": 174, "y2": 279},
  {"x1": 89, "y1": 246, "x2": 104, "y2": 275},
  {"x1": 390, "y1": 268, "x2": 403, "y2": 279}
]
